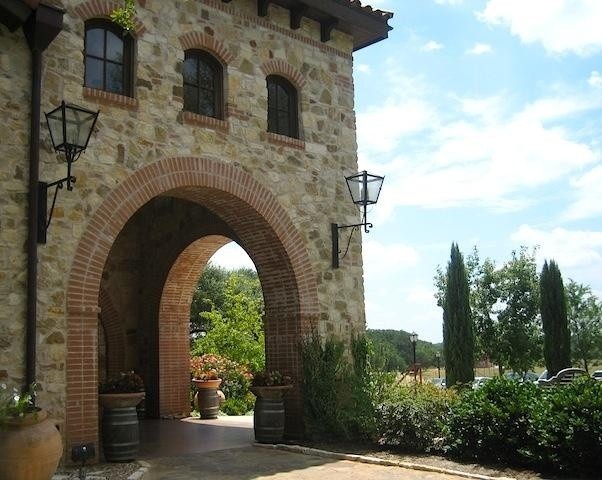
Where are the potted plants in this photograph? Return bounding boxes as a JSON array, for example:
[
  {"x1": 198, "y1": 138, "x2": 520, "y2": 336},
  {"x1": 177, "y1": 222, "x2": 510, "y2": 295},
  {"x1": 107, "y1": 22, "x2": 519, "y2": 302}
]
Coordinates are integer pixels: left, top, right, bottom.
[
  {"x1": 247, "y1": 367, "x2": 293, "y2": 443},
  {"x1": 98, "y1": 370, "x2": 146, "y2": 462},
  {"x1": 192, "y1": 368, "x2": 222, "y2": 419}
]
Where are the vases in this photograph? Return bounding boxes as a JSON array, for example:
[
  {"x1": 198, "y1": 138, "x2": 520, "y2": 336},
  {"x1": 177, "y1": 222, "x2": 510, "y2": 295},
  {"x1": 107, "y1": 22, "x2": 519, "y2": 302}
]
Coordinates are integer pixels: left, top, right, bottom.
[{"x1": 0, "y1": 407, "x2": 64, "y2": 480}]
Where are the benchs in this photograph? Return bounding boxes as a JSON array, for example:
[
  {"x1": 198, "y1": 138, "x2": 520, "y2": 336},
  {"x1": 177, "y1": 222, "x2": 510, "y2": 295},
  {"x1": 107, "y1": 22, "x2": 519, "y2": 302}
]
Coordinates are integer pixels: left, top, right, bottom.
[{"x1": 537, "y1": 368, "x2": 588, "y2": 394}]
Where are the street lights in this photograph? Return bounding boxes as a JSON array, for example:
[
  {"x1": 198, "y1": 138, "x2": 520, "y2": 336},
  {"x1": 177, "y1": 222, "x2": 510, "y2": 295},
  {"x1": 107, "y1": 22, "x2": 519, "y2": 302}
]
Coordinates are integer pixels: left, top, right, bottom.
[
  {"x1": 436, "y1": 352, "x2": 440, "y2": 378},
  {"x1": 408, "y1": 331, "x2": 418, "y2": 380}
]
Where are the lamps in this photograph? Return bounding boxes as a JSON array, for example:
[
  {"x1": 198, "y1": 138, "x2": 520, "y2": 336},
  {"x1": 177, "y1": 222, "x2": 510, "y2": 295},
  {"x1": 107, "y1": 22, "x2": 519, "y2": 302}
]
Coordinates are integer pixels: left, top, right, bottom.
[
  {"x1": 37, "y1": 100, "x2": 101, "y2": 243},
  {"x1": 331, "y1": 170, "x2": 386, "y2": 268}
]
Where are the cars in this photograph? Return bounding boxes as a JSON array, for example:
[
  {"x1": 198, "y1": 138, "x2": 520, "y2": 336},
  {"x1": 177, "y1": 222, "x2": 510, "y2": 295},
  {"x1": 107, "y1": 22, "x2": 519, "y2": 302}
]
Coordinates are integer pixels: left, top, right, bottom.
[{"x1": 432, "y1": 370, "x2": 602, "y2": 391}]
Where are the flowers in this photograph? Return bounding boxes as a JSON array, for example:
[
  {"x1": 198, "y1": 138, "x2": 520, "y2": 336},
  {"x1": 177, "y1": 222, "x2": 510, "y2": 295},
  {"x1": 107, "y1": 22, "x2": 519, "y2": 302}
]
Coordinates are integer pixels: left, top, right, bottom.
[{"x1": 0, "y1": 382, "x2": 39, "y2": 422}]
